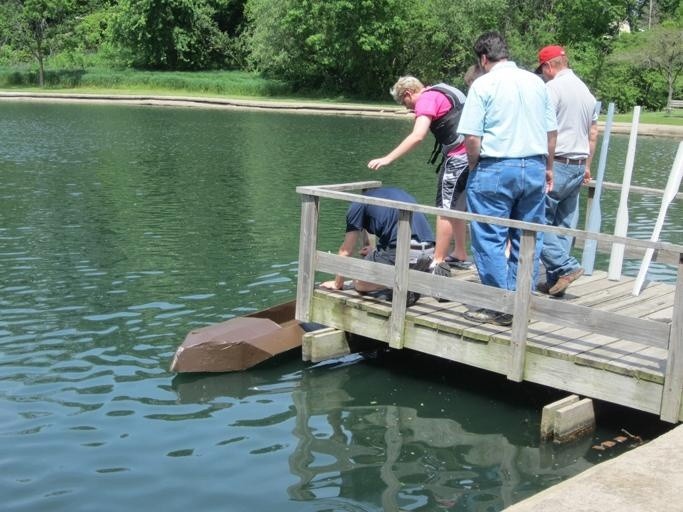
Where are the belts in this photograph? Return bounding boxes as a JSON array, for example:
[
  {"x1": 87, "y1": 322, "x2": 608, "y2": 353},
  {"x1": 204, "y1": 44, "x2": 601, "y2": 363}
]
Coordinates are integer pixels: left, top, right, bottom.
[
  {"x1": 389, "y1": 243, "x2": 433, "y2": 250},
  {"x1": 554, "y1": 156, "x2": 586, "y2": 165}
]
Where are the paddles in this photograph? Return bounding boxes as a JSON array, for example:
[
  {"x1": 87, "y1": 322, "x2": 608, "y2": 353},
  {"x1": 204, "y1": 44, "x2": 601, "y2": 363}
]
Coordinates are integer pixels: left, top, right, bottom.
[
  {"x1": 566, "y1": 193, "x2": 579, "y2": 256},
  {"x1": 632, "y1": 142, "x2": 683, "y2": 296},
  {"x1": 607, "y1": 105, "x2": 640, "y2": 281},
  {"x1": 580, "y1": 103, "x2": 614, "y2": 276}
]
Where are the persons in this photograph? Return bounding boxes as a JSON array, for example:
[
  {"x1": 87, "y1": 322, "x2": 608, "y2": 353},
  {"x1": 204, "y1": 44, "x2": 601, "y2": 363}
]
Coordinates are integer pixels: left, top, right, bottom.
[
  {"x1": 317, "y1": 179, "x2": 437, "y2": 305},
  {"x1": 457, "y1": 32, "x2": 558, "y2": 326},
  {"x1": 366, "y1": 78, "x2": 468, "y2": 268},
  {"x1": 463, "y1": 61, "x2": 485, "y2": 89},
  {"x1": 533, "y1": 43, "x2": 600, "y2": 298}
]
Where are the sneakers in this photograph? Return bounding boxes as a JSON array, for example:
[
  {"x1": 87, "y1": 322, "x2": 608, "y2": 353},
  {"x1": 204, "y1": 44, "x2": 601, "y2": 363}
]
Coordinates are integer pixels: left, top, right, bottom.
[
  {"x1": 464, "y1": 309, "x2": 513, "y2": 326},
  {"x1": 538, "y1": 268, "x2": 584, "y2": 297},
  {"x1": 406, "y1": 291, "x2": 420, "y2": 307},
  {"x1": 434, "y1": 256, "x2": 472, "y2": 302}
]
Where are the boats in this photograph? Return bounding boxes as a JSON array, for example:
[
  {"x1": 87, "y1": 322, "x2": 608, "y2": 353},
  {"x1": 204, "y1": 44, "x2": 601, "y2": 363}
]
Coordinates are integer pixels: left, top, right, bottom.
[{"x1": 168, "y1": 297, "x2": 331, "y2": 375}]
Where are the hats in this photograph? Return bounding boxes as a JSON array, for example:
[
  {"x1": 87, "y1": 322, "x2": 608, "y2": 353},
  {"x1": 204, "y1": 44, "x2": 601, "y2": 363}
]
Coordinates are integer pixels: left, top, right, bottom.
[{"x1": 534, "y1": 46, "x2": 566, "y2": 74}]
